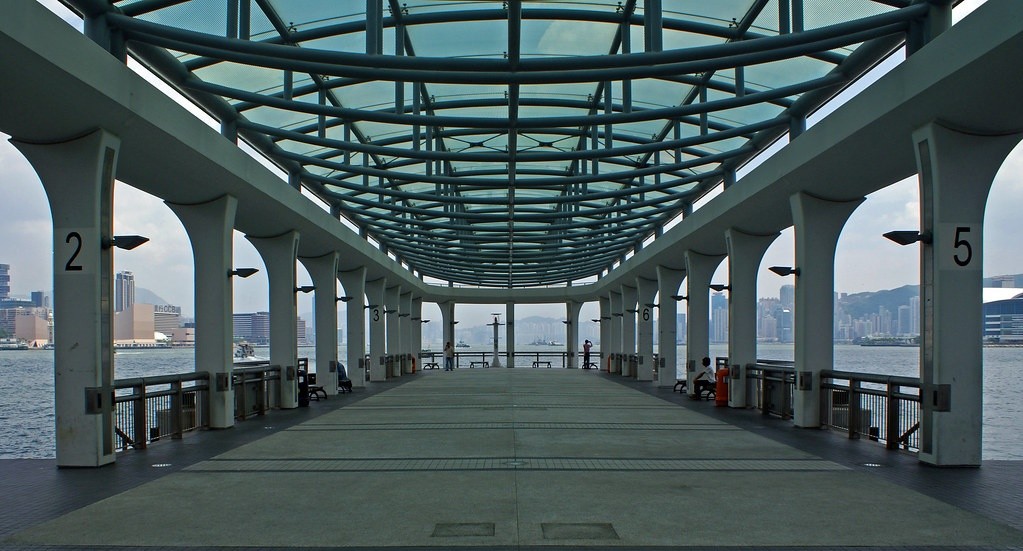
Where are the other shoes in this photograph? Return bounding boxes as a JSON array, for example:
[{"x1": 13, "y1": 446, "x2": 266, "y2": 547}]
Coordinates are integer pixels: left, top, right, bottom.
[
  {"x1": 689, "y1": 393, "x2": 701, "y2": 400},
  {"x1": 451, "y1": 369, "x2": 453, "y2": 370},
  {"x1": 445, "y1": 369, "x2": 449, "y2": 371},
  {"x1": 584, "y1": 367, "x2": 591, "y2": 369}
]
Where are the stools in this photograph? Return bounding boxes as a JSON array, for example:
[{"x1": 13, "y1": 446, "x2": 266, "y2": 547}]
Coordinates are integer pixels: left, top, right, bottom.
[{"x1": 338, "y1": 379, "x2": 349, "y2": 393}]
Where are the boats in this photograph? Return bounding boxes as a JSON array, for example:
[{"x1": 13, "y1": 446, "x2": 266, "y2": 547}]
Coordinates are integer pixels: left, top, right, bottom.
[
  {"x1": 233, "y1": 343, "x2": 270, "y2": 367},
  {"x1": 549, "y1": 340, "x2": 564, "y2": 346},
  {"x1": 455, "y1": 339, "x2": 470, "y2": 348}
]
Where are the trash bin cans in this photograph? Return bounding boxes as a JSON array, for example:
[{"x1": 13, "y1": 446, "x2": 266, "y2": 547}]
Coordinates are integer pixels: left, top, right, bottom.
[
  {"x1": 297, "y1": 370, "x2": 310, "y2": 408},
  {"x1": 714, "y1": 368, "x2": 729, "y2": 407},
  {"x1": 412, "y1": 356, "x2": 416, "y2": 374}
]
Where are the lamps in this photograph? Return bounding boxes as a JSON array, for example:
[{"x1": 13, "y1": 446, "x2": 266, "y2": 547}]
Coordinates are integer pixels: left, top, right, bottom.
[
  {"x1": 451, "y1": 321, "x2": 459, "y2": 324},
  {"x1": 294, "y1": 285, "x2": 316, "y2": 293},
  {"x1": 335, "y1": 296, "x2": 353, "y2": 303},
  {"x1": 507, "y1": 321, "x2": 512, "y2": 325},
  {"x1": 228, "y1": 268, "x2": 259, "y2": 278},
  {"x1": 882, "y1": 230, "x2": 932, "y2": 245},
  {"x1": 363, "y1": 303, "x2": 430, "y2": 323},
  {"x1": 592, "y1": 295, "x2": 690, "y2": 322},
  {"x1": 101, "y1": 235, "x2": 149, "y2": 250},
  {"x1": 769, "y1": 267, "x2": 800, "y2": 276},
  {"x1": 562, "y1": 321, "x2": 571, "y2": 324},
  {"x1": 707, "y1": 284, "x2": 732, "y2": 292}
]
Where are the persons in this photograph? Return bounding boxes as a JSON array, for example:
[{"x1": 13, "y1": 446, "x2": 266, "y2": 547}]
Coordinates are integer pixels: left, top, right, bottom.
[
  {"x1": 338, "y1": 362, "x2": 352, "y2": 392},
  {"x1": 444, "y1": 342, "x2": 454, "y2": 371},
  {"x1": 688, "y1": 357, "x2": 715, "y2": 401},
  {"x1": 583, "y1": 339, "x2": 593, "y2": 369}
]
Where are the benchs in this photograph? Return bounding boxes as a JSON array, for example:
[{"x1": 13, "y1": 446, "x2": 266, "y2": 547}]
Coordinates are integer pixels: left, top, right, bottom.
[
  {"x1": 674, "y1": 380, "x2": 687, "y2": 393},
  {"x1": 470, "y1": 362, "x2": 490, "y2": 368},
  {"x1": 531, "y1": 362, "x2": 551, "y2": 369},
  {"x1": 307, "y1": 374, "x2": 328, "y2": 402},
  {"x1": 422, "y1": 363, "x2": 440, "y2": 369},
  {"x1": 582, "y1": 363, "x2": 599, "y2": 370},
  {"x1": 696, "y1": 373, "x2": 718, "y2": 401}
]
